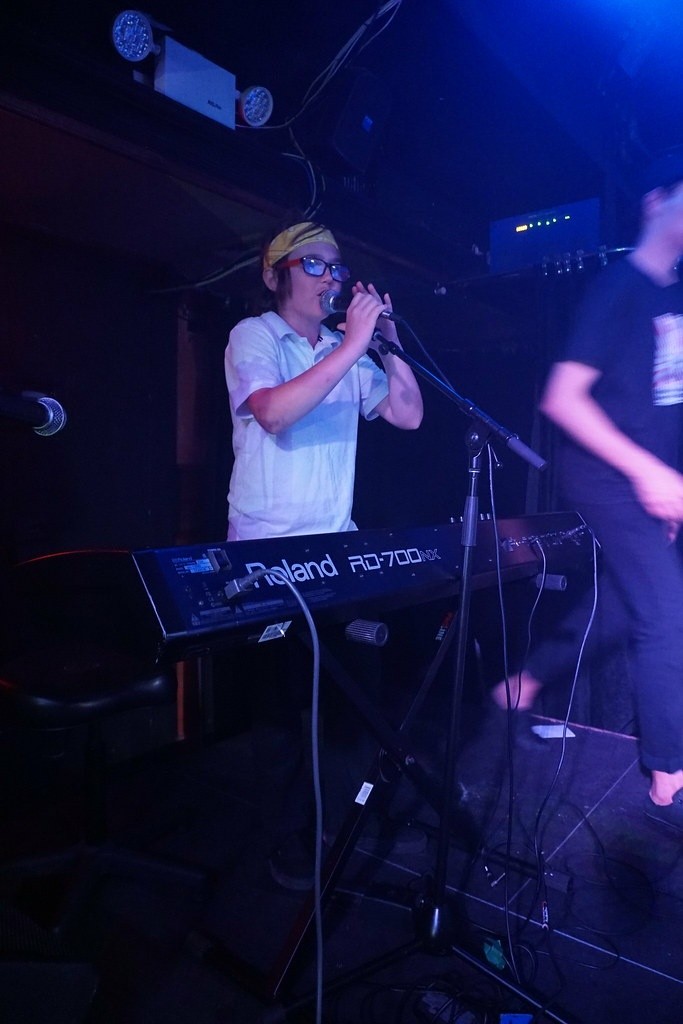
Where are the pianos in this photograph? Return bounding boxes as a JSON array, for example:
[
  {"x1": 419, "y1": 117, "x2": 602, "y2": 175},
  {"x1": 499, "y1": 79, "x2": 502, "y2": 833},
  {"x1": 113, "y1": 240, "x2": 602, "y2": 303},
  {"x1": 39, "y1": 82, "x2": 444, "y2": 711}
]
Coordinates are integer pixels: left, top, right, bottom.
[{"x1": 129, "y1": 507, "x2": 608, "y2": 1006}]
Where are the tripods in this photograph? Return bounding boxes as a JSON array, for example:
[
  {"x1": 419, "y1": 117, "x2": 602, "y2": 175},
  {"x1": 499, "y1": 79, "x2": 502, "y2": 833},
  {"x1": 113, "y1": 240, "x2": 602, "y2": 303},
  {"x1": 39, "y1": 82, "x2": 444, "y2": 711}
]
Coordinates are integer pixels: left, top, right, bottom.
[{"x1": 265, "y1": 321, "x2": 572, "y2": 1024}]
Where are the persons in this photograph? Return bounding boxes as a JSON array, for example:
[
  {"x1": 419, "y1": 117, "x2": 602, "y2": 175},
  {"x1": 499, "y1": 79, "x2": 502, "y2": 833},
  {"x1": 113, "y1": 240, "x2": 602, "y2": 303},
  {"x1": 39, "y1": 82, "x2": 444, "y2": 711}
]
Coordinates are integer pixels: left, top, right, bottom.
[
  {"x1": 224, "y1": 217, "x2": 423, "y2": 891},
  {"x1": 491, "y1": 150, "x2": 683, "y2": 839}
]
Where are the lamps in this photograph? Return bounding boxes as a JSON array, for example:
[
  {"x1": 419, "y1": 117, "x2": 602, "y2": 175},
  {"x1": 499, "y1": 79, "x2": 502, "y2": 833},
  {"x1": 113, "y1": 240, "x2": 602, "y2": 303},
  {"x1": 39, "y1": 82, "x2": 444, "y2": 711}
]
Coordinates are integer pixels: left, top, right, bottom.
[{"x1": 106, "y1": 10, "x2": 274, "y2": 134}]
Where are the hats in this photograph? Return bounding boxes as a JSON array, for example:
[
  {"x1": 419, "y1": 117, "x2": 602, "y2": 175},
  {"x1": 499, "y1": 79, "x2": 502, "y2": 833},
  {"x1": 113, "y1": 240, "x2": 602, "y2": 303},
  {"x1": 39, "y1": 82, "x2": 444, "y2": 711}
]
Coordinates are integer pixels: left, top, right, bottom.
[{"x1": 263, "y1": 219, "x2": 339, "y2": 267}]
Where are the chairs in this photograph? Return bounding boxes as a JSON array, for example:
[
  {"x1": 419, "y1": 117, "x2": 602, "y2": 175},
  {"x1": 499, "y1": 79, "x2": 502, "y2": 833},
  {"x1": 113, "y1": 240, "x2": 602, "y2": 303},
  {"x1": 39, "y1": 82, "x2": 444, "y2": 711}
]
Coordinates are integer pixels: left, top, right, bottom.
[{"x1": 0, "y1": 547, "x2": 217, "y2": 947}]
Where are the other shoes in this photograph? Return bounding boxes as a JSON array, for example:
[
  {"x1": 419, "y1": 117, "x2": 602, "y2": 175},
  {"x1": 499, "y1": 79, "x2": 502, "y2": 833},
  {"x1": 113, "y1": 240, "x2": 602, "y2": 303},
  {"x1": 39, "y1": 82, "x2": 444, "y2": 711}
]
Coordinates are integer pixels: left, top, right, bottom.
[{"x1": 643, "y1": 786, "x2": 683, "y2": 838}]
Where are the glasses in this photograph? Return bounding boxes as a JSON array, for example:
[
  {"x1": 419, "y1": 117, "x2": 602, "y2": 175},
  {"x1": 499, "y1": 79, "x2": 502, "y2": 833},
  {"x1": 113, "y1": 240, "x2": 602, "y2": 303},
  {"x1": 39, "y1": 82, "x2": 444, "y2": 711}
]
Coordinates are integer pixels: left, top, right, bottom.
[{"x1": 277, "y1": 257, "x2": 351, "y2": 282}]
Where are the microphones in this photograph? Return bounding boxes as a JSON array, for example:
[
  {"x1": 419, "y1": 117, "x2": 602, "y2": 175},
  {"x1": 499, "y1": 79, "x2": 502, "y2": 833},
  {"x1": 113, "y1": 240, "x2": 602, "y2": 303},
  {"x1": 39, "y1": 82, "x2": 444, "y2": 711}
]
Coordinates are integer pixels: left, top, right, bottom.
[
  {"x1": 0, "y1": 395, "x2": 68, "y2": 436},
  {"x1": 320, "y1": 289, "x2": 401, "y2": 322}
]
